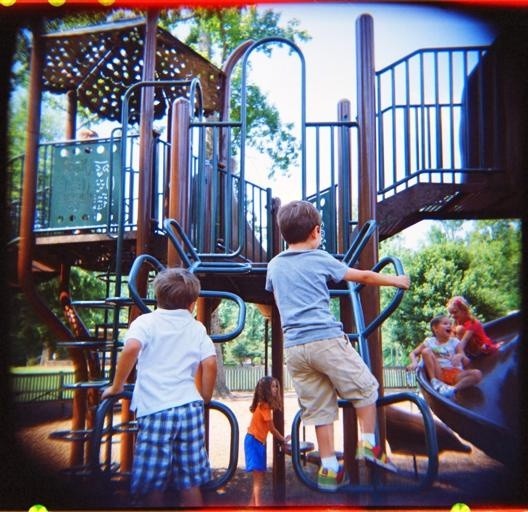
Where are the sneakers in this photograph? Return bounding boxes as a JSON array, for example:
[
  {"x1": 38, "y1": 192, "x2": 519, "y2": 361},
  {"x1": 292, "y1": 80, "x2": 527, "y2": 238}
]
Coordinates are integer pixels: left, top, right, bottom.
[
  {"x1": 317, "y1": 464, "x2": 350, "y2": 490},
  {"x1": 355, "y1": 439, "x2": 398, "y2": 473},
  {"x1": 432, "y1": 378, "x2": 456, "y2": 400}
]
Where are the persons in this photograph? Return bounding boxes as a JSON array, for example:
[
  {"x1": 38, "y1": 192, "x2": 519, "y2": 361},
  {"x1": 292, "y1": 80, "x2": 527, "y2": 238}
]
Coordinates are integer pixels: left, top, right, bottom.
[
  {"x1": 265, "y1": 200, "x2": 412, "y2": 492},
  {"x1": 100, "y1": 267, "x2": 219, "y2": 507},
  {"x1": 244, "y1": 375, "x2": 292, "y2": 506},
  {"x1": 445, "y1": 296, "x2": 494, "y2": 359},
  {"x1": 406, "y1": 314, "x2": 482, "y2": 400},
  {"x1": 66, "y1": 129, "x2": 101, "y2": 234}
]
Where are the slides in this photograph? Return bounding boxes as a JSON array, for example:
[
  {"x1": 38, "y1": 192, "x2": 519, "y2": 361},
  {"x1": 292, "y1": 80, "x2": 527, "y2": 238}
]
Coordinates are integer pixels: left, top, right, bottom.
[{"x1": 416, "y1": 312, "x2": 521, "y2": 468}]
